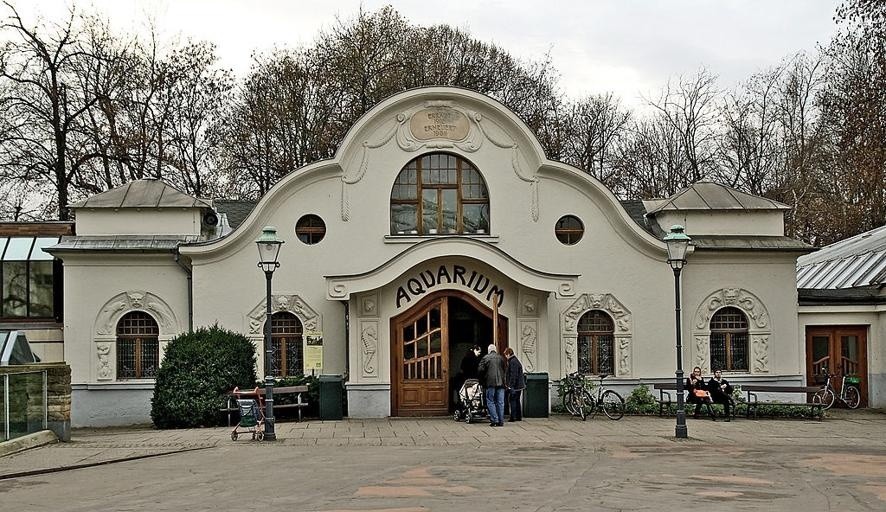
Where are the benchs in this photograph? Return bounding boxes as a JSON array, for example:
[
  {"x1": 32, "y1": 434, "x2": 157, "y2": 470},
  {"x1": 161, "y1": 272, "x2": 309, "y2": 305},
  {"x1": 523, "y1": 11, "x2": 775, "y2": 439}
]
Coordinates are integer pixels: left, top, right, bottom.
[
  {"x1": 654, "y1": 383, "x2": 738, "y2": 420},
  {"x1": 741, "y1": 385, "x2": 829, "y2": 421},
  {"x1": 219, "y1": 384, "x2": 309, "y2": 427}
]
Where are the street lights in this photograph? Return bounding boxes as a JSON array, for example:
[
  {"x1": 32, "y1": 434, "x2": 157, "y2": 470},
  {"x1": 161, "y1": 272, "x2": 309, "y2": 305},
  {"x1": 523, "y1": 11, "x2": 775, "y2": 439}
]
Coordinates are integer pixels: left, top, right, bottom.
[
  {"x1": 663, "y1": 223, "x2": 692, "y2": 439},
  {"x1": 255, "y1": 225, "x2": 286, "y2": 440}
]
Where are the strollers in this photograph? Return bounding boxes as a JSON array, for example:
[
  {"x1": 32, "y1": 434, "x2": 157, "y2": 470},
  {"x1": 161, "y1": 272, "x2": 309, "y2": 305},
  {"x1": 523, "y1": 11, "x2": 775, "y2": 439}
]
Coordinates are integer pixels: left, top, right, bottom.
[
  {"x1": 453, "y1": 377, "x2": 489, "y2": 424},
  {"x1": 230, "y1": 385, "x2": 266, "y2": 442}
]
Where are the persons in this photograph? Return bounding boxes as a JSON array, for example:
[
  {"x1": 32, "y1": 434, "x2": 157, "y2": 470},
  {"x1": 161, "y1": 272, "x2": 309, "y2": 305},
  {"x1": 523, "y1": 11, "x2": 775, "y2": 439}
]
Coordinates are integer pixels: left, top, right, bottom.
[
  {"x1": 706, "y1": 367, "x2": 735, "y2": 423},
  {"x1": 476, "y1": 344, "x2": 507, "y2": 426},
  {"x1": 95, "y1": 342, "x2": 112, "y2": 378},
  {"x1": 502, "y1": 347, "x2": 526, "y2": 422},
  {"x1": 685, "y1": 366, "x2": 717, "y2": 423},
  {"x1": 458, "y1": 344, "x2": 481, "y2": 418},
  {"x1": 467, "y1": 384, "x2": 481, "y2": 407}
]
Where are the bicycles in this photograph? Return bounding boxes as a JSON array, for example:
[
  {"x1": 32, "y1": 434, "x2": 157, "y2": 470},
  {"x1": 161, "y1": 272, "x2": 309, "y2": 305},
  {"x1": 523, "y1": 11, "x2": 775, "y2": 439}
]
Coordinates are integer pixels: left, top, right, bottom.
[
  {"x1": 563, "y1": 371, "x2": 587, "y2": 421},
  {"x1": 568, "y1": 373, "x2": 626, "y2": 421},
  {"x1": 812, "y1": 367, "x2": 861, "y2": 411}
]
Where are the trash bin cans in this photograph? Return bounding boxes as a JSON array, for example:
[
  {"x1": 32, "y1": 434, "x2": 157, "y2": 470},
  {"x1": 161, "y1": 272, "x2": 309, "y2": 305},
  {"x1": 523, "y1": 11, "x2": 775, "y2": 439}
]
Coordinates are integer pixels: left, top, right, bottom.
[
  {"x1": 523, "y1": 373, "x2": 549, "y2": 418},
  {"x1": 319, "y1": 374, "x2": 343, "y2": 421}
]
